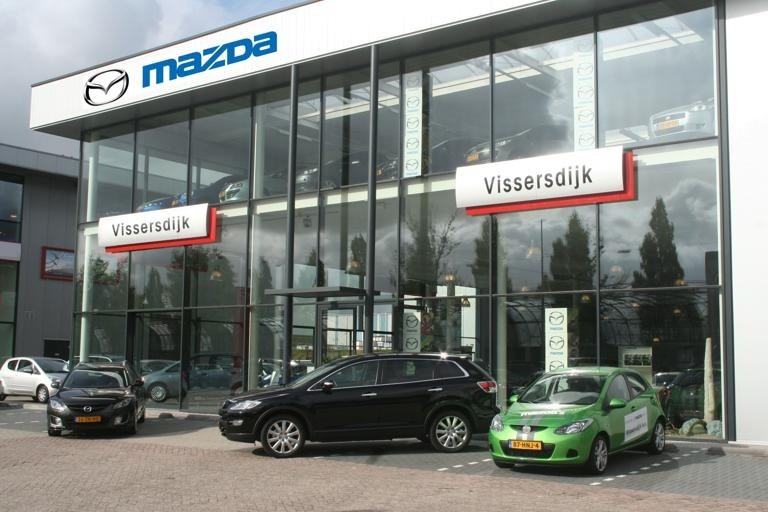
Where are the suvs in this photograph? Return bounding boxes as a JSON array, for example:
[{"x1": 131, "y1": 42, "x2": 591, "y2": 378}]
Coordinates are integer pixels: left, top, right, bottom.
[{"x1": 216, "y1": 349, "x2": 501, "y2": 461}]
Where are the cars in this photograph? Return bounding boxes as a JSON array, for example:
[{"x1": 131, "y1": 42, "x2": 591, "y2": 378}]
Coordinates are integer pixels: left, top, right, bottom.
[
  {"x1": 505, "y1": 355, "x2": 723, "y2": 427},
  {"x1": 485, "y1": 364, "x2": 669, "y2": 477},
  {"x1": 646, "y1": 96, "x2": 714, "y2": 138},
  {"x1": 72, "y1": 351, "x2": 316, "y2": 404},
  {"x1": 46, "y1": 360, "x2": 147, "y2": 438},
  {"x1": 0, "y1": 355, "x2": 70, "y2": 404}
]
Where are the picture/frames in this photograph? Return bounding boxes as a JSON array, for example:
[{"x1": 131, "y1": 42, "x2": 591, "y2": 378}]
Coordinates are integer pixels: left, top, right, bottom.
[{"x1": 40, "y1": 245, "x2": 120, "y2": 285}]
